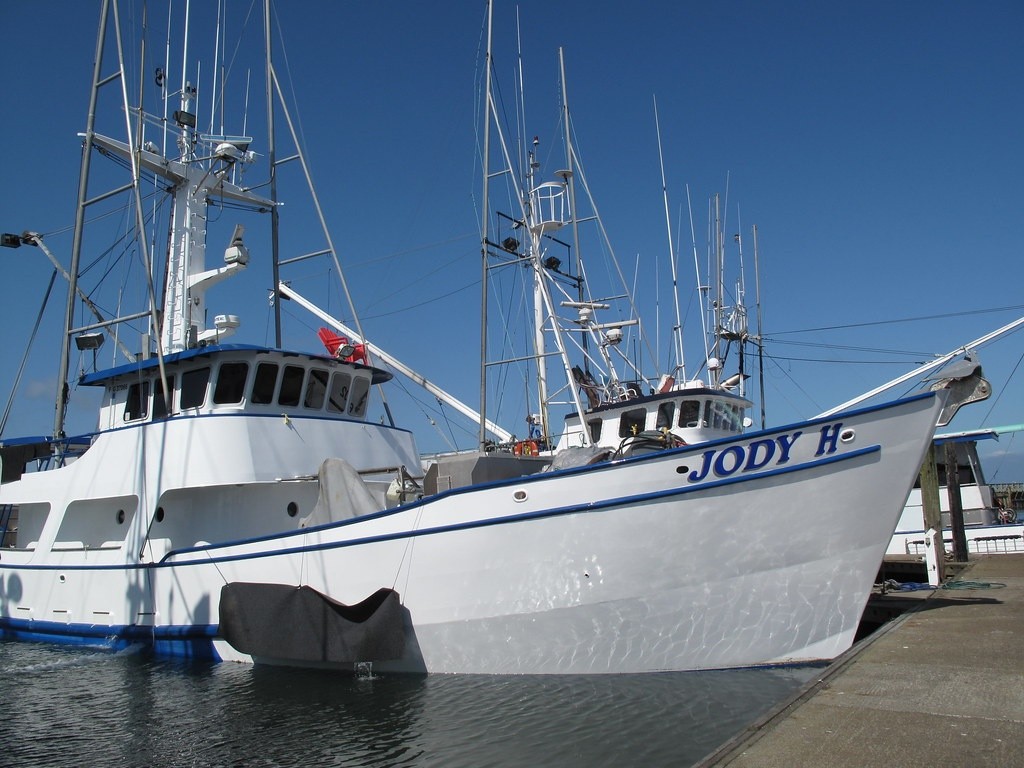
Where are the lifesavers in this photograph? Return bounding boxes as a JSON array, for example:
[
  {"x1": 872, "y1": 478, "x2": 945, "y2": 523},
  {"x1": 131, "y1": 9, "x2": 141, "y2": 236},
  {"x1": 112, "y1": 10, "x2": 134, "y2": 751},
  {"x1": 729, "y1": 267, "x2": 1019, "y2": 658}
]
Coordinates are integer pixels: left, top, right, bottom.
[{"x1": 515, "y1": 441, "x2": 539, "y2": 457}]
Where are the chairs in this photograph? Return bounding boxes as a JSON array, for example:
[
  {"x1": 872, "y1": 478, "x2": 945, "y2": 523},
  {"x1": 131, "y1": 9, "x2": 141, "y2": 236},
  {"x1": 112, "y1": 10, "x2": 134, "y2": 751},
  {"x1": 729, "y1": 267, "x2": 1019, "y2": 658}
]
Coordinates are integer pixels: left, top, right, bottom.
[{"x1": 318, "y1": 327, "x2": 368, "y2": 366}]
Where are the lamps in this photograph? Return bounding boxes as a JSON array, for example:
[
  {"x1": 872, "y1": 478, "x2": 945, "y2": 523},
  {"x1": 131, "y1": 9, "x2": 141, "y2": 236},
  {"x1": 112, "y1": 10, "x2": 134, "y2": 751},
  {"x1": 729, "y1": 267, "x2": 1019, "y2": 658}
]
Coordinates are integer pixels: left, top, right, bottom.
[
  {"x1": 336, "y1": 344, "x2": 355, "y2": 361},
  {"x1": 0, "y1": 233, "x2": 24, "y2": 248},
  {"x1": 172, "y1": 109, "x2": 198, "y2": 128},
  {"x1": 502, "y1": 237, "x2": 520, "y2": 252},
  {"x1": 544, "y1": 256, "x2": 560, "y2": 269},
  {"x1": 75, "y1": 332, "x2": 104, "y2": 372}
]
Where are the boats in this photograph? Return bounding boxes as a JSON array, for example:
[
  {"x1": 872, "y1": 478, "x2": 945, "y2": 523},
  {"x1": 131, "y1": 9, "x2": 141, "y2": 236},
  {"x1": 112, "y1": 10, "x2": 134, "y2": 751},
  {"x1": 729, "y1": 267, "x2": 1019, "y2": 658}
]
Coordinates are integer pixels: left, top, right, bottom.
[
  {"x1": 1, "y1": 0, "x2": 992, "y2": 673},
  {"x1": 418, "y1": 0, "x2": 1023, "y2": 560}
]
